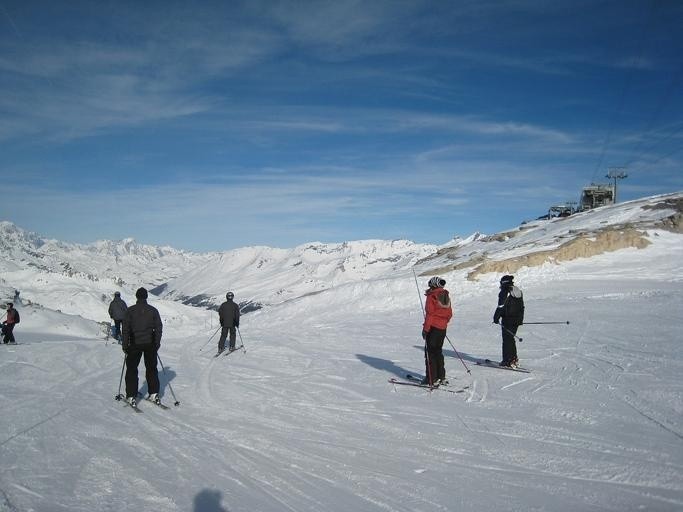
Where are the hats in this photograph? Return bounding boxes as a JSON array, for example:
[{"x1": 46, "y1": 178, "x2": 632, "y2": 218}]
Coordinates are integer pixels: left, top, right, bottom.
[
  {"x1": 500, "y1": 275, "x2": 514, "y2": 287},
  {"x1": 428, "y1": 277, "x2": 446, "y2": 288},
  {"x1": 136, "y1": 287, "x2": 148, "y2": 299}
]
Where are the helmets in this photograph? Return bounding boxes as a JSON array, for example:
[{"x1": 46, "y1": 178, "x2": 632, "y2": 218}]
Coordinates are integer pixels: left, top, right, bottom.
[{"x1": 226, "y1": 292, "x2": 234, "y2": 299}]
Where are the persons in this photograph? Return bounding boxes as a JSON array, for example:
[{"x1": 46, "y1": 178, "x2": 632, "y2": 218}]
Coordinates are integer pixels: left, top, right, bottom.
[
  {"x1": 422, "y1": 277, "x2": 452, "y2": 384},
  {"x1": 1, "y1": 303, "x2": 16, "y2": 344},
  {"x1": 109, "y1": 292, "x2": 127, "y2": 340},
  {"x1": 218, "y1": 292, "x2": 239, "y2": 353},
  {"x1": 122, "y1": 288, "x2": 162, "y2": 402},
  {"x1": 494, "y1": 275, "x2": 524, "y2": 366}
]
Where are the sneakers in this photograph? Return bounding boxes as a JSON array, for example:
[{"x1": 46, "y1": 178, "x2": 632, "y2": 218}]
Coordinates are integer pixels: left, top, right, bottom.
[
  {"x1": 218, "y1": 347, "x2": 225, "y2": 353},
  {"x1": 126, "y1": 392, "x2": 136, "y2": 405},
  {"x1": 499, "y1": 359, "x2": 518, "y2": 367},
  {"x1": 229, "y1": 345, "x2": 235, "y2": 351},
  {"x1": 421, "y1": 377, "x2": 439, "y2": 386},
  {"x1": 143, "y1": 392, "x2": 161, "y2": 402}
]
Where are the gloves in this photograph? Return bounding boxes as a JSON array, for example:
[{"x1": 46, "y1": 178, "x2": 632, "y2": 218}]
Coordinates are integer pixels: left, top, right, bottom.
[
  {"x1": 152, "y1": 344, "x2": 160, "y2": 352},
  {"x1": 422, "y1": 330, "x2": 429, "y2": 339},
  {"x1": 494, "y1": 314, "x2": 500, "y2": 324},
  {"x1": 122, "y1": 344, "x2": 129, "y2": 354}
]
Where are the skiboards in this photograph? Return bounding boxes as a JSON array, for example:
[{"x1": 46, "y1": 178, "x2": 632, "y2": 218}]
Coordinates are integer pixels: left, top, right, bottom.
[
  {"x1": 478, "y1": 359, "x2": 532, "y2": 374},
  {"x1": 215, "y1": 344, "x2": 242, "y2": 358},
  {"x1": 120, "y1": 392, "x2": 172, "y2": 413},
  {"x1": 388, "y1": 375, "x2": 469, "y2": 393}
]
